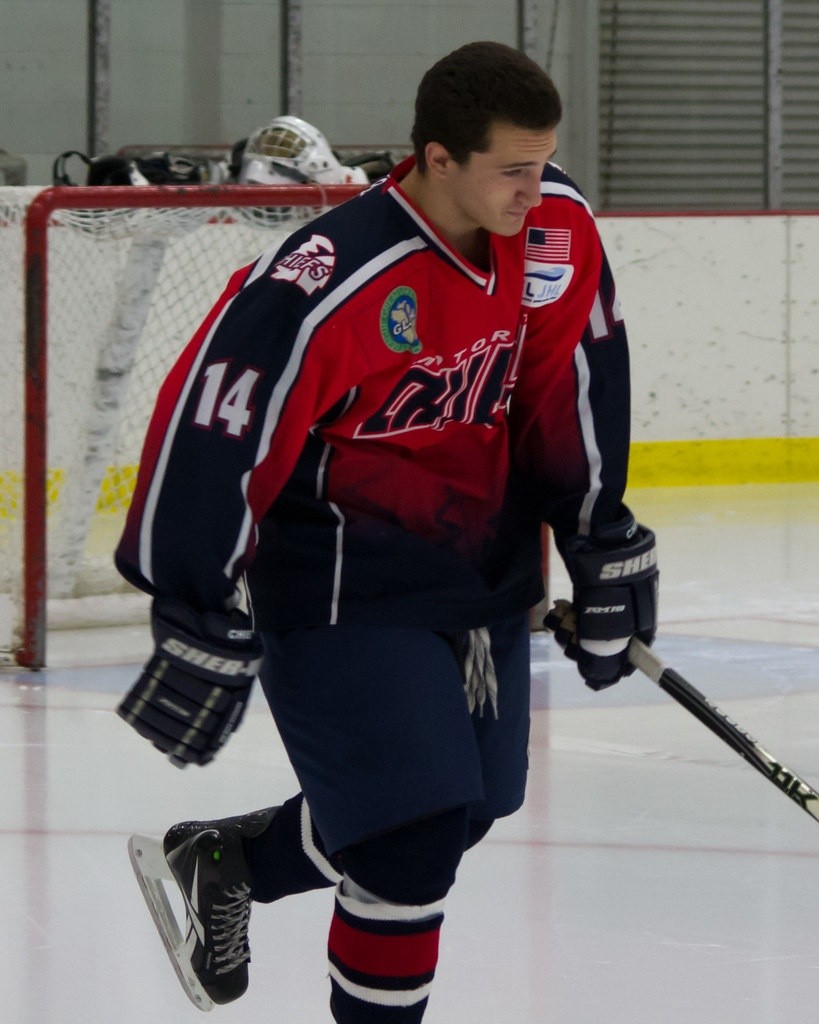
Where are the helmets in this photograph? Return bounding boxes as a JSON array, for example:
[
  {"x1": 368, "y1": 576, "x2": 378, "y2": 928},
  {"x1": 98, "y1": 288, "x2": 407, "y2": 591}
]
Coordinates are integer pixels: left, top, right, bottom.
[{"x1": 241, "y1": 116, "x2": 368, "y2": 185}]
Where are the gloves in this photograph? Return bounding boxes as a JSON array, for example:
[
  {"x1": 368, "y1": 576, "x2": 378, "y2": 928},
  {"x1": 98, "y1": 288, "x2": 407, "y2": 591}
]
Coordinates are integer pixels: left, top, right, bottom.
[
  {"x1": 117, "y1": 600, "x2": 263, "y2": 770},
  {"x1": 542, "y1": 500, "x2": 659, "y2": 690}
]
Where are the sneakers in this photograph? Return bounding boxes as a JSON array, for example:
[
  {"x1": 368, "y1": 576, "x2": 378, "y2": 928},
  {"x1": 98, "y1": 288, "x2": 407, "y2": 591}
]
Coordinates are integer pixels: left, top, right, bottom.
[{"x1": 127, "y1": 805, "x2": 281, "y2": 1013}]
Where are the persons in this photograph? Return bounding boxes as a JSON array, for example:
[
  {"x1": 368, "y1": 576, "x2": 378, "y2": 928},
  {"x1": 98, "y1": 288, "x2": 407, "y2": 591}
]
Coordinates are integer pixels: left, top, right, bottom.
[{"x1": 114, "y1": 37, "x2": 659, "y2": 1024}]
